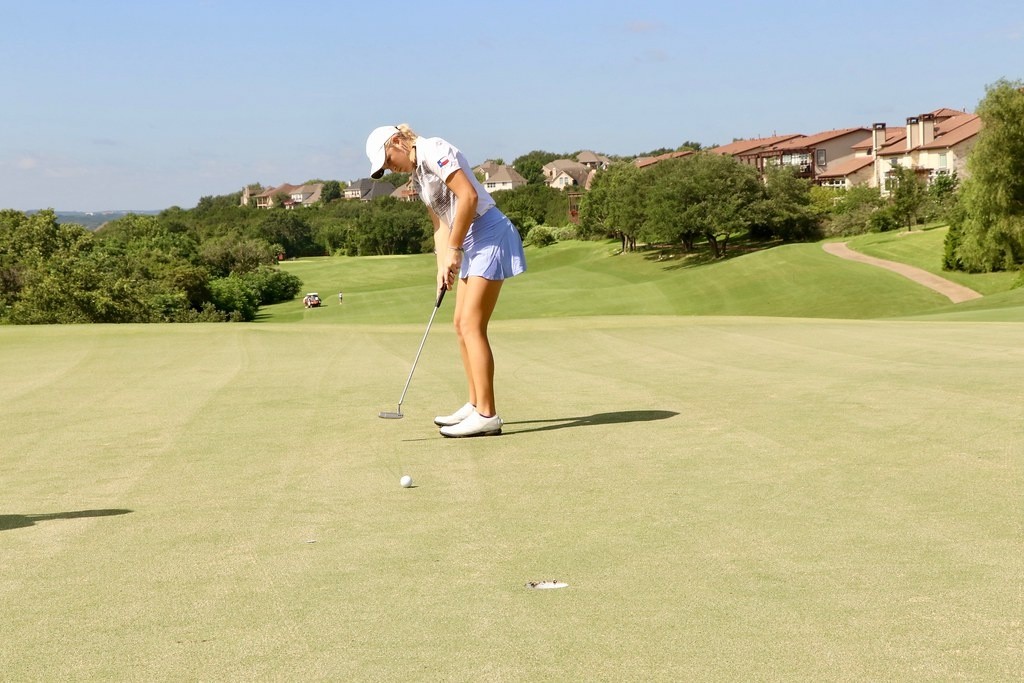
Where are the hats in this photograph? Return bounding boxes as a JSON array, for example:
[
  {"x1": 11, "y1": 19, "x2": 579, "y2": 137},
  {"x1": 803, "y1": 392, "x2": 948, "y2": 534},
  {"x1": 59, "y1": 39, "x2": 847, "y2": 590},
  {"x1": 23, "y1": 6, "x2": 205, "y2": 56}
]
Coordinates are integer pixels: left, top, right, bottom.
[{"x1": 366, "y1": 126, "x2": 399, "y2": 179}]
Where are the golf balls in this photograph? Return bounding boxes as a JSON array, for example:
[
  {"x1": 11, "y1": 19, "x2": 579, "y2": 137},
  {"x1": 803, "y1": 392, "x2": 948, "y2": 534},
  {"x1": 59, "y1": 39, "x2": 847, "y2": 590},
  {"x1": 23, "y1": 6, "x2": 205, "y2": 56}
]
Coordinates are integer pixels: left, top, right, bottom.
[{"x1": 401, "y1": 475, "x2": 412, "y2": 488}]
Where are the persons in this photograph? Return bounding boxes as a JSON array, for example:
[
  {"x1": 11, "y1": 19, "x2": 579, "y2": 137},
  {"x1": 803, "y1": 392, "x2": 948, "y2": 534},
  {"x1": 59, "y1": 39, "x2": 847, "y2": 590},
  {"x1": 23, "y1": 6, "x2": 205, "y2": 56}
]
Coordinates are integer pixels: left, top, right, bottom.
[
  {"x1": 339, "y1": 292, "x2": 343, "y2": 305},
  {"x1": 303, "y1": 295, "x2": 321, "y2": 309},
  {"x1": 366, "y1": 124, "x2": 527, "y2": 437}
]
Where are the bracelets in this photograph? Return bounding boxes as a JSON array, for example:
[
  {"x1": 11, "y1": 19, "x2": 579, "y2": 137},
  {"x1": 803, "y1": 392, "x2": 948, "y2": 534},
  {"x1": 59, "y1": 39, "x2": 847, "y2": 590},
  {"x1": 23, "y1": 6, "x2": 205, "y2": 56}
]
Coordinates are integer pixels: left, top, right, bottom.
[{"x1": 447, "y1": 246, "x2": 465, "y2": 253}]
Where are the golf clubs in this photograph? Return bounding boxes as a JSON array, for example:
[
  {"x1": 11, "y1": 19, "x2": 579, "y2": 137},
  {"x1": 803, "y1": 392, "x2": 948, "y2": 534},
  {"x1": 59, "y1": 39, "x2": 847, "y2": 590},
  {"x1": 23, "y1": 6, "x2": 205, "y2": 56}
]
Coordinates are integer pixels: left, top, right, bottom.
[{"x1": 377, "y1": 269, "x2": 454, "y2": 420}]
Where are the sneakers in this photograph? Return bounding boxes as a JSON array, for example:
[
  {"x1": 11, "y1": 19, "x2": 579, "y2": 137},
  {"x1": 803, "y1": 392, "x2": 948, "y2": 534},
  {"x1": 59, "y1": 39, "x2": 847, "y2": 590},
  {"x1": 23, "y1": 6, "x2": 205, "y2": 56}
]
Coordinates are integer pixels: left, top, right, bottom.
[
  {"x1": 434, "y1": 402, "x2": 476, "y2": 425},
  {"x1": 440, "y1": 411, "x2": 504, "y2": 438}
]
